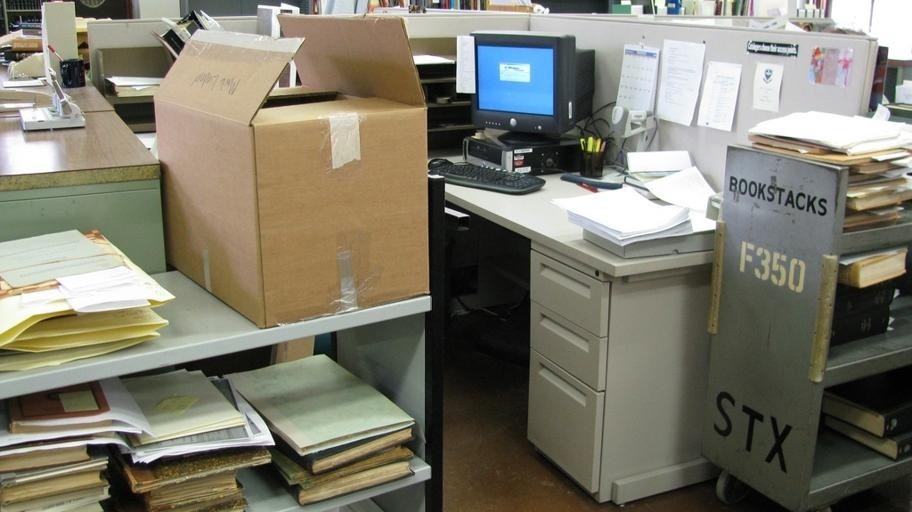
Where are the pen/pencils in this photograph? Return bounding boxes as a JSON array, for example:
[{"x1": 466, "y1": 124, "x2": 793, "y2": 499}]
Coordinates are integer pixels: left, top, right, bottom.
[
  {"x1": 576, "y1": 181, "x2": 597, "y2": 192},
  {"x1": 48, "y1": 44, "x2": 64, "y2": 62},
  {"x1": 580, "y1": 136, "x2": 607, "y2": 176}
]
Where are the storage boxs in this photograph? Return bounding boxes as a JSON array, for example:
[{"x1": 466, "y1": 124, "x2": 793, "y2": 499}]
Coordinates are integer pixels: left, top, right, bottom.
[{"x1": 150, "y1": 12, "x2": 430, "y2": 331}]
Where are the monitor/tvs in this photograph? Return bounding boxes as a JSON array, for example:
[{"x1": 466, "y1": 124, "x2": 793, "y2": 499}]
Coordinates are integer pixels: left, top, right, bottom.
[{"x1": 469, "y1": 30, "x2": 596, "y2": 147}]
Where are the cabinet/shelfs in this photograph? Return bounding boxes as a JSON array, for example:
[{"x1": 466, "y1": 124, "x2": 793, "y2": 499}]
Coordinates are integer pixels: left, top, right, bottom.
[
  {"x1": 0, "y1": 171, "x2": 444, "y2": 511},
  {"x1": 702, "y1": 142, "x2": 911, "y2": 512},
  {"x1": 409, "y1": 37, "x2": 486, "y2": 157},
  {"x1": 93, "y1": 47, "x2": 175, "y2": 134}
]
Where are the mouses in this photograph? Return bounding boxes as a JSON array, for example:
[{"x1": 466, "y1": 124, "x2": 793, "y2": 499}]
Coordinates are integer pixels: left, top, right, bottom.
[{"x1": 428, "y1": 159, "x2": 454, "y2": 169}]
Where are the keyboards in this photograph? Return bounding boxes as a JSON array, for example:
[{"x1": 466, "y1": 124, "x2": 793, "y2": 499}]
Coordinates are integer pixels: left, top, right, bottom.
[{"x1": 428, "y1": 163, "x2": 547, "y2": 196}]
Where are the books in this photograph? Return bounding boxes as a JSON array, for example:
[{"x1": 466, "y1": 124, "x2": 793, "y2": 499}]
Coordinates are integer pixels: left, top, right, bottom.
[
  {"x1": 821, "y1": 365, "x2": 911, "y2": 460},
  {"x1": 749, "y1": 136, "x2": 911, "y2": 348},
  {"x1": 0, "y1": 352, "x2": 416, "y2": 510}
]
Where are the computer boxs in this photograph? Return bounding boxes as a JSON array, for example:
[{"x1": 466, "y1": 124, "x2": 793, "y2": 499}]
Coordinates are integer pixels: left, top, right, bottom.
[{"x1": 461, "y1": 132, "x2": 589, "y2": 173}]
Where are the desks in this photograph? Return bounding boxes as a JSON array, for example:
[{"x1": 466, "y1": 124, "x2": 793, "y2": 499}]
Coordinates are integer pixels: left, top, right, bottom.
[
  {"x1": 428, "y1": 145, "x2": 721, "y2": 509},
  {"x1": 0, "y1": 60, "x2": 167, "y2": 275}
]
